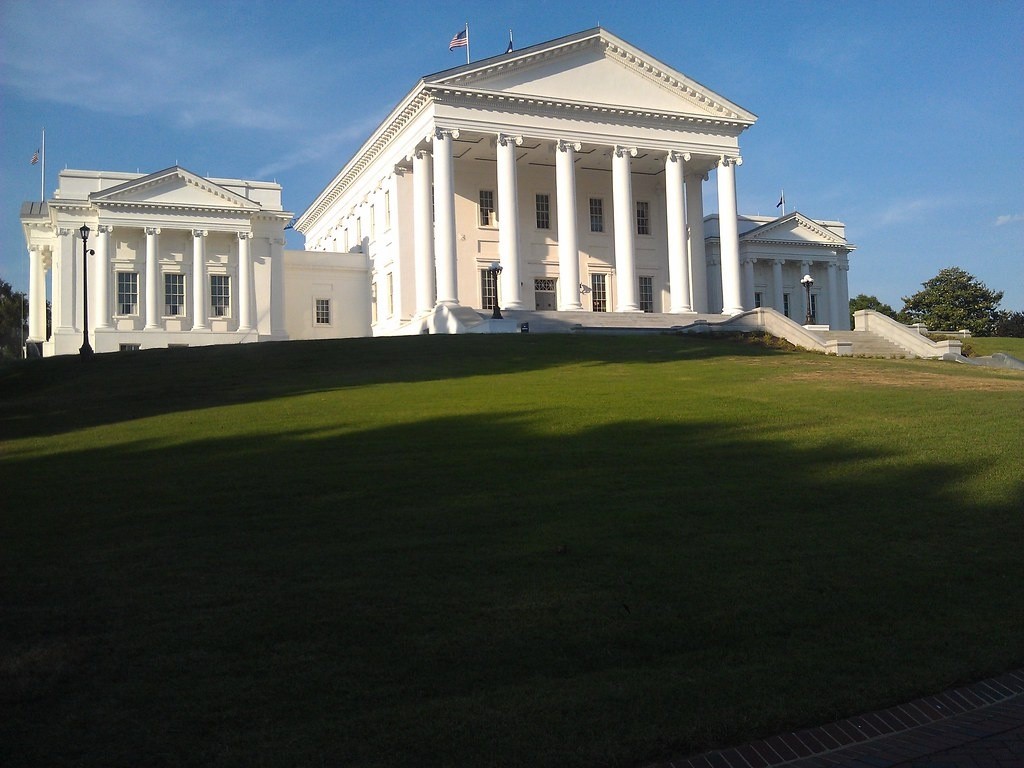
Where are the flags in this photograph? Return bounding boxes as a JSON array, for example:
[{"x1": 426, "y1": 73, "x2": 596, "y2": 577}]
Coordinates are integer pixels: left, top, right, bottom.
[
  {"x1": 449, "y1": 30, "x2": 467, "y2": 51},
  {"x1": 505, "y1": 41, "x2": 513, "y2": 54},
  {"x1": 31, "y1": 148, "x2": 39, "y2": 165},
  {"x1": 777, "y1": 195, "x2": 786, "y2": 208}
]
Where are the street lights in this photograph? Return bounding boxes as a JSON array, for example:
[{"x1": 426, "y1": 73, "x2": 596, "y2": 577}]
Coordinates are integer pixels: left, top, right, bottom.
[
  {"x1": 78, "y1": 221, "x2": 95, "y2": 355},
  {"x1": 800, "y1": 275, "x2": 814, "y2": 326},
  {"x1": 488, "y1": 261, "x2": 504, "y2": 319}
]
